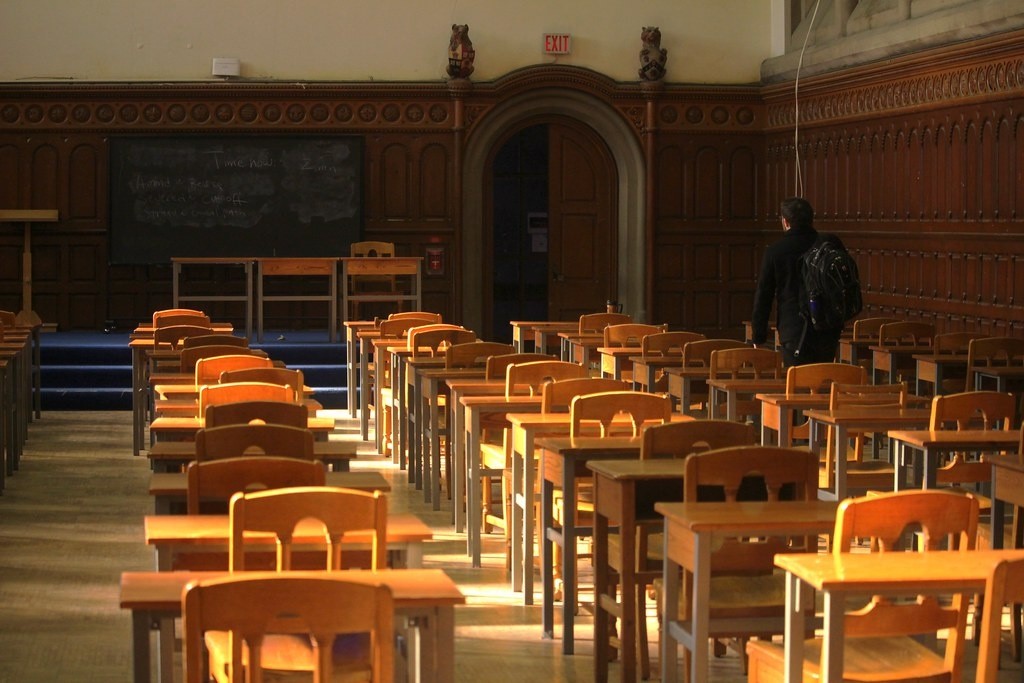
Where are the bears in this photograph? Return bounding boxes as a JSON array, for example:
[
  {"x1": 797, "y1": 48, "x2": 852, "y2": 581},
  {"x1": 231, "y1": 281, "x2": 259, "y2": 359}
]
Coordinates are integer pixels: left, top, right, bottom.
[
  {"x1": 445, "y1": 23, "x2": 475, "y2": 81},
  {"x1": 638, "y1": 25, "x2": 669, "y2": 80}
]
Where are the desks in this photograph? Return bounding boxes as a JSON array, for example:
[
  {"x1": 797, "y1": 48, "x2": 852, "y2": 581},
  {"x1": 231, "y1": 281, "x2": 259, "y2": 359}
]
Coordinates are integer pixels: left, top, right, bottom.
[
  {"x1": 0, "y1": 324, "x2": 42, "y2": 494},
  {"x1": 120, "y1": 255, "x2": 1024, "y2": 683}
]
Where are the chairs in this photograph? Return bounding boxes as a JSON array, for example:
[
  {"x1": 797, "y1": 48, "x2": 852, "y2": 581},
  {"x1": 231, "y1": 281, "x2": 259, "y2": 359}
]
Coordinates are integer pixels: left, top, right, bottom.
[{"x1": 151, "y1": 240, "x2": 1023, "y2": 683}]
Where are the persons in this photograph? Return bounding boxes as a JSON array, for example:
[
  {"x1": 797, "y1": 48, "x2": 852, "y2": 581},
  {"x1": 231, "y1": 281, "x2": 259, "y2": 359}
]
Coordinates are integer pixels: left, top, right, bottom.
[{"x1": 751, "y1": 197, "x2": 846, "y2": 446}]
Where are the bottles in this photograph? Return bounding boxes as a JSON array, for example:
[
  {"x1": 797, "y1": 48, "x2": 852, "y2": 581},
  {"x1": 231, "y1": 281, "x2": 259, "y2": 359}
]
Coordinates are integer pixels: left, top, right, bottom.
[
  {"x1": 425, "y1": 238, "x2": 444, "y2": 275},
  {"x1": 809, "y1": 289, "x2": 817, "y2": 308}
]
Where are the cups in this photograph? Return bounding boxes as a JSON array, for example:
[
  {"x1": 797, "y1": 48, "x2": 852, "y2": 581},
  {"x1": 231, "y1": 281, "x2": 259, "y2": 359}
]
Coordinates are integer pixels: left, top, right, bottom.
[{"x1": 607, "y1": 300, "x2": 623, "y2": 314}]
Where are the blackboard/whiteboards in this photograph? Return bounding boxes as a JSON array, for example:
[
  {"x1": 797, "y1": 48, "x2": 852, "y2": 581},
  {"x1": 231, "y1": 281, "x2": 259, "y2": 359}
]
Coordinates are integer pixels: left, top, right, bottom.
[{"x1": 107, "y1": 133, "x2": 365, "y2": 267}]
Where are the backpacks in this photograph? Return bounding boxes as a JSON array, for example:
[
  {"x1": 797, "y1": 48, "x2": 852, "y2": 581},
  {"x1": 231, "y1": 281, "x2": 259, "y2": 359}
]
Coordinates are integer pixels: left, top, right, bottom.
[{"x1": 793, "y1": 240, "x2": 865, "y2": 323}]
